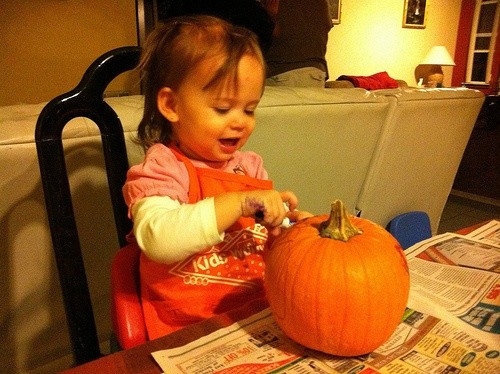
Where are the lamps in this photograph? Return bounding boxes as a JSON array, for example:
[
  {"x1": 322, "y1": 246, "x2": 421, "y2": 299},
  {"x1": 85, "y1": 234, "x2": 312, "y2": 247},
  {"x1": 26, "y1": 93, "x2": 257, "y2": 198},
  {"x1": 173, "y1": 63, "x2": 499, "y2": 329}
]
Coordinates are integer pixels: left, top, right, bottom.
[{"x1": 418, "y1": 46, "x2": 456, "y2": 87}]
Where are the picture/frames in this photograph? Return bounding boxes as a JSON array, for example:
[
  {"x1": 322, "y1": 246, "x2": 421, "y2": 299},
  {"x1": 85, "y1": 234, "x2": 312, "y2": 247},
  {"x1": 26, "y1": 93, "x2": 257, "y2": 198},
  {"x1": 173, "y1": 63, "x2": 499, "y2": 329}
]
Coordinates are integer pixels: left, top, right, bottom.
[
  {"x1": 402, "y1": 0, "x2": 428, "y2": 28},
  {"x1": 328, "y1": 0, "x2": 343, "y2": 25}
]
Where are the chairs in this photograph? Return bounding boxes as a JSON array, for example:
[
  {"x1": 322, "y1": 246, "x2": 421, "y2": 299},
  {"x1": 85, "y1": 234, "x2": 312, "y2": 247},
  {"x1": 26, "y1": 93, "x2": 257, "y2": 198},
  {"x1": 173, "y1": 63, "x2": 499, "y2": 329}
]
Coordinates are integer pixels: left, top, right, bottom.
[{"x1": 34, "y1": 46, "x2": 173, "y2": 368}]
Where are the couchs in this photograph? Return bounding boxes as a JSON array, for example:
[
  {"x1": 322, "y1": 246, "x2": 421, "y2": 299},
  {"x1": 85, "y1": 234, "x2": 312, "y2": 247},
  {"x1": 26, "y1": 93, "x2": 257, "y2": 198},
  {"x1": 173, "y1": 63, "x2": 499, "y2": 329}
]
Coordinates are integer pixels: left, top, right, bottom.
[
  {"x1": 0, "y1": 66, "x2": 487, "y2": 374},
  {"x1": 448, "y1": 94, "x2": 500, "y2": 209},
  {"x1": 324, "y1": 70, "x2": 409, "y2": 91}
]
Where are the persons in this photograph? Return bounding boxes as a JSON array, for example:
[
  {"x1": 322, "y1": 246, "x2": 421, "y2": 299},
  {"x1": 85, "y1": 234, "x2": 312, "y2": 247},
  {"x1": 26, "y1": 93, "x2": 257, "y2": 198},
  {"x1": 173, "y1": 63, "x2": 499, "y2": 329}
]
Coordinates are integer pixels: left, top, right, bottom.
[
  {"x1": 158, "y1": 0, "x2": 335, "y2": 88},
  {"x1": 121, "y1": 16, "x2": 314, "y2": 340}
]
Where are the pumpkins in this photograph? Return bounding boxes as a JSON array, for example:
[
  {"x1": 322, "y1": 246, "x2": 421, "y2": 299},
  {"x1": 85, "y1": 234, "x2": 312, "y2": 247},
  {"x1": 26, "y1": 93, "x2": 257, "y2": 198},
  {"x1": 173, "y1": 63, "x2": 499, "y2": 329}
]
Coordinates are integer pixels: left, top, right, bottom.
[{"x1": 265, "y1": 200, "x2": 410, "y2": 357}]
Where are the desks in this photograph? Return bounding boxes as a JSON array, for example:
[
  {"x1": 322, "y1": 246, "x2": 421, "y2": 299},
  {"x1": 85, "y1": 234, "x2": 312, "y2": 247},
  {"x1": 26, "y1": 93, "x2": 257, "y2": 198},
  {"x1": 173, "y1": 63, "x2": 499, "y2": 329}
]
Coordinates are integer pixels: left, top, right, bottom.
[{"x1": 60, "y1": 217, "x2": 500, "y2": 374}]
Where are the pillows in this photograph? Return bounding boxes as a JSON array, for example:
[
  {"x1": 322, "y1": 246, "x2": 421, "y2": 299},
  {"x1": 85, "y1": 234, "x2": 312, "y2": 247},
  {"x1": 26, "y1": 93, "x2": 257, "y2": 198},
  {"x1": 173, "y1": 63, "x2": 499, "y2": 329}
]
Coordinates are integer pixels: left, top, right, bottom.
[{"x1": 265, "y1": 67, "x2": 327, "y2": 89}]
